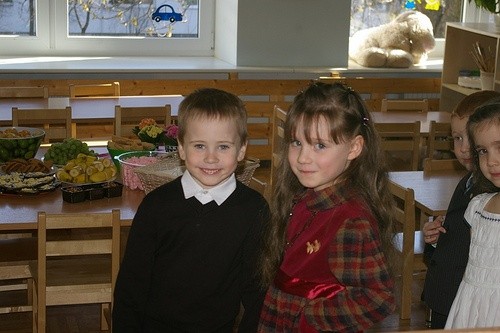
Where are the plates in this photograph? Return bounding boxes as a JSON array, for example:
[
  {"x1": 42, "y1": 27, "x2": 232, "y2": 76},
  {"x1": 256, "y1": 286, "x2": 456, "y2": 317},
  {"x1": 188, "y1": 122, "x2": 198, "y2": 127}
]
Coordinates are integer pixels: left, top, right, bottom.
[
  {"x1": 41, "y1": 156, "x2": 100, "y2": 167},
  {"x1": 53, "y1": 174, "x2": 117, "y2": 185}
]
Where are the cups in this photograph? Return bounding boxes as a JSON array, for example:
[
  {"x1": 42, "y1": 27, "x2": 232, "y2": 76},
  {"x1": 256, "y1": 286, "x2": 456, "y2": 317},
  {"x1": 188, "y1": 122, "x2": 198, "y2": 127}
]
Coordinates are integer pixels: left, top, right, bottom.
[{"x1": 480, "y1": 68, "x2": 493, "y2": 91}]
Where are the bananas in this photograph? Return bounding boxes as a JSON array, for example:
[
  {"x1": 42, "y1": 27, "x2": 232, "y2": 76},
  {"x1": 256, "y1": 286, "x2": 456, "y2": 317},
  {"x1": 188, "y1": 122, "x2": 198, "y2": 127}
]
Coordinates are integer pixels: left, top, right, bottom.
[{"x1": 58, "y1": 153, "x2": 117, "y2": 184}]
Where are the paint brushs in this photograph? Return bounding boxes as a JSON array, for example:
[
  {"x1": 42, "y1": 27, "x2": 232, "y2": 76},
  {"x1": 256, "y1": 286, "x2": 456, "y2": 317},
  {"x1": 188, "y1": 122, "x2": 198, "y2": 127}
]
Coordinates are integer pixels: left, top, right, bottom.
[{"x1": 470, "y1": 39, "x2": 496, "y2": 74}]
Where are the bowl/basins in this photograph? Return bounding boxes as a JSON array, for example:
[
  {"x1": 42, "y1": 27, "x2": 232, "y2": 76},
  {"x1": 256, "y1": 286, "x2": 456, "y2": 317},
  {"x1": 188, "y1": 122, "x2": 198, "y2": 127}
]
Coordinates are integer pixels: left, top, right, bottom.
[
  {"x1": 114, "y1": 151, "x2": 168, "y2": 192},
  {"x1": 0, "y1": 126, "x2": 45, "y2": 160}
]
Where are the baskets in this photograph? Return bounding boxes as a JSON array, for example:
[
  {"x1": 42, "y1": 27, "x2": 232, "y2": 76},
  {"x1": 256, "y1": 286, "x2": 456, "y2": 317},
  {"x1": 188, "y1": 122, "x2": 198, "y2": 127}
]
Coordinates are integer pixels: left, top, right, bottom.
[
  {"x1": 116, "y1": 150, "x2": 173, "y2": 190},
  {"x1": 134, "y1": 152, "x2": 261, "y2": 196}
]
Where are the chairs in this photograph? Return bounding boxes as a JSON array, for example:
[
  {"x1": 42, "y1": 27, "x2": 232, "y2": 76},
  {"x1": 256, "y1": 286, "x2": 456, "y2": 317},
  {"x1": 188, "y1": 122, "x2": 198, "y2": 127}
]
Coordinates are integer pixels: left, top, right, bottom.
[
  {"x1": 13, "y1": 107, "x2": 73, "y2": 145},
  {"x1": 429, "y1": 120, "x2": 462, "y2": 160},
  {"x1": 1, "y1": 85, "x2": 49, "y2": 145},
  {"x1": 269, "y1": 102, "x2": 290, "y2": 191},
  {"x1": 27, "y1": 210, "x2": 121, "y2": 333},
  {"x1": 420, "y1": 158, "x2": 466, "y2": 235},
  {"x1": 382, "y1": 179, "x2": 429, "y2": 321},
  {"x1": 365, "y1": 120, "x2": 419, "y2": 178},
  {"x1": 113, "y1": 103, "x2": 173, "y2": 136},
  {"x1": 381, "y1": 98, "x2": 429, "y2": 115},
  {"x1": 70, "y1": 82, "x2": 121, "y2": 139}
]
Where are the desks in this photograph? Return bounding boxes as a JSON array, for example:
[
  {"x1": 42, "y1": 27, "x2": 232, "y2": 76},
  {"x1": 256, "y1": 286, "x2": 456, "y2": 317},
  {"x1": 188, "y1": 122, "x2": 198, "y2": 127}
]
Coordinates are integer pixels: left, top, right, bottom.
[
  {"x1": 0, "y1": 93, "x2": 184, "y2": 141},
  {"x1": 384, "y1": 171, "x2": 470, "y2": 234}
]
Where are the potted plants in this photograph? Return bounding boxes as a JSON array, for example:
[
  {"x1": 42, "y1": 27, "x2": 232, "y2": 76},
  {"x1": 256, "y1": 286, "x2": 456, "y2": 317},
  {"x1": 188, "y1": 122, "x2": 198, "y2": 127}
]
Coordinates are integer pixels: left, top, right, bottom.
[{"x1": 466, "y1": 0, "x2": 499, "y2": 28}]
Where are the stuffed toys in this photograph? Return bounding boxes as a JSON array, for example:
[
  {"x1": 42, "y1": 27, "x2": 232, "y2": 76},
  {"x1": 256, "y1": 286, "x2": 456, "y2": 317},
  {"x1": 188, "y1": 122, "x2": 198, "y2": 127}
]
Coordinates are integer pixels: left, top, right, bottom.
[{"x1": 348, "y1": 10, "x2": 437, "y2": 68}]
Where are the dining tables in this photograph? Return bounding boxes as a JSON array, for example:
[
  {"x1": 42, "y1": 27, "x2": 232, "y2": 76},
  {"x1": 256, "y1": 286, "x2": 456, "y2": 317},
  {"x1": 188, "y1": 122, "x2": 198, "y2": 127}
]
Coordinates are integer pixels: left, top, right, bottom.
[{"x1": 0, "y1": 144, "x2": 148, "y2": 313}]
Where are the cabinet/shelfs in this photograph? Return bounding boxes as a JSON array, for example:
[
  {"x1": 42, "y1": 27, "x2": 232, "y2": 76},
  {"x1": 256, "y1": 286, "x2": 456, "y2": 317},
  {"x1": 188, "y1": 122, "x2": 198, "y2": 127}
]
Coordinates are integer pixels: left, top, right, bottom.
[{"x1": 438, "y1": 23, "x2": 500, "y2": 128}]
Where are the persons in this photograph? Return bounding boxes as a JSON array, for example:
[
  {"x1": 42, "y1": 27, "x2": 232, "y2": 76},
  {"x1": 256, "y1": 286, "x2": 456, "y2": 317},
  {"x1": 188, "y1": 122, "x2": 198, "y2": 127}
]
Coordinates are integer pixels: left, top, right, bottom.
[
  {"x1": 420, "y1": 91, "x2": 500, "y2": 328},
  {"x1": 111, "y1": 89, "x2": 271, "y2": 333},
  {"x1": 256, "y1": 82, "x2": 402, "y2": 333},
  {"x1": 445, "y1": 103, "x2": 500, "y2": 333}
]
